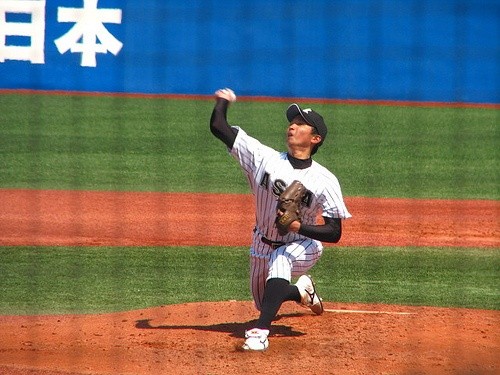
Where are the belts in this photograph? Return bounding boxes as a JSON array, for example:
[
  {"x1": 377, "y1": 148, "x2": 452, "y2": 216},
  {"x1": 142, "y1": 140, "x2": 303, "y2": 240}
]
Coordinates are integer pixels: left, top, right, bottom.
[{"x1": 253, "y1": 227, "x2": 284, "y2": 249}]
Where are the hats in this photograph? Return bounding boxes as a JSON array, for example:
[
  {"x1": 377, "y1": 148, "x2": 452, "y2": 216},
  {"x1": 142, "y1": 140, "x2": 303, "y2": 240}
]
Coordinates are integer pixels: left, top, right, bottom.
[{"x1": 285, "y1": 103, "x2": 328, "y2": 142}]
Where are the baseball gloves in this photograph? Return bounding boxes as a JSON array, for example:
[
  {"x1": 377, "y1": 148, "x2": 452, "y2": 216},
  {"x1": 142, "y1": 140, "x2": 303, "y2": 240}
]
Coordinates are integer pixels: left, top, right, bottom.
[{"x1": 275, "y1": 180, "x2": 314, "y2": 236}]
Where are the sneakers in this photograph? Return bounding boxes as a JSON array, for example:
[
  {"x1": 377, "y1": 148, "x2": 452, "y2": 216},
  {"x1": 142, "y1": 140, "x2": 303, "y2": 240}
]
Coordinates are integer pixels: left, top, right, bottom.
[
  {"x1": 239, "y1": 327, "x2": 269, "y2": 351},
  {"x1": 295, "y1": 273, "x2": 324, "y2": 315}
]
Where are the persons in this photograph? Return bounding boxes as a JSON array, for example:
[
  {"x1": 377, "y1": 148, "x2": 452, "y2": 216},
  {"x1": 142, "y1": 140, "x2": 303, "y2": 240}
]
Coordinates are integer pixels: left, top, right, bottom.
[{"x1": 210, "y1": 88, "x2": 353, "y2": 352}]
